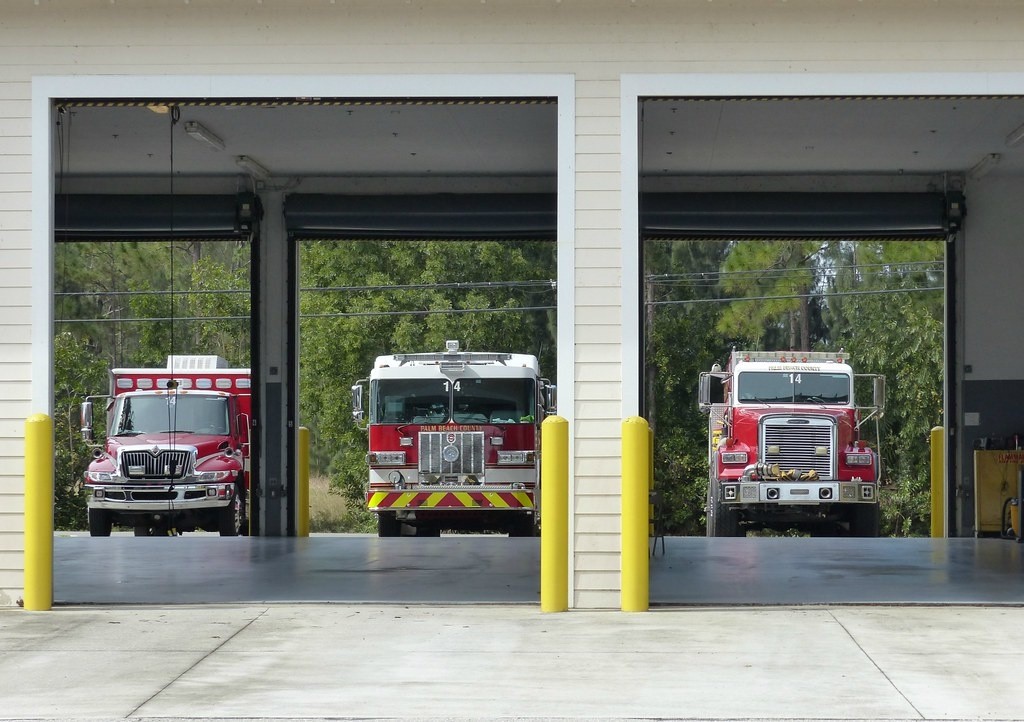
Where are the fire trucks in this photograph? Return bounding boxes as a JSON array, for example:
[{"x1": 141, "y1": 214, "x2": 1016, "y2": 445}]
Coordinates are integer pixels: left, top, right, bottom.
[
  {"x1": 76, "y1": 357, "x2": 252, "y2": 540},
  {"x1": 351, "y1": 340, "x2": 556, "y2": 538},
  {"x1": 698, "y1": 345, "x2": 886, "y2": 537}
]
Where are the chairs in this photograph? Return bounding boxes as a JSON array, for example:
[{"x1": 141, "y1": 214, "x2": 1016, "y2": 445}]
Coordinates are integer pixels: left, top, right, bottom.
[{"x1": 489, "y1": 409, "x2": 526, "y2": 423}]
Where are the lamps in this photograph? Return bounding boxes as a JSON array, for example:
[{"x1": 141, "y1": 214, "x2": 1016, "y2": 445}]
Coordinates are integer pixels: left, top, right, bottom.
[
  {"x1": 184, "y1": 122, "x2": 224, "y2": 152},
  {"x1": 968, "y1": 154, "x2": 1000, "y2": 180},
  {"x1": 236, "y1": 155, "x2": 270, "y2": 178}
]
